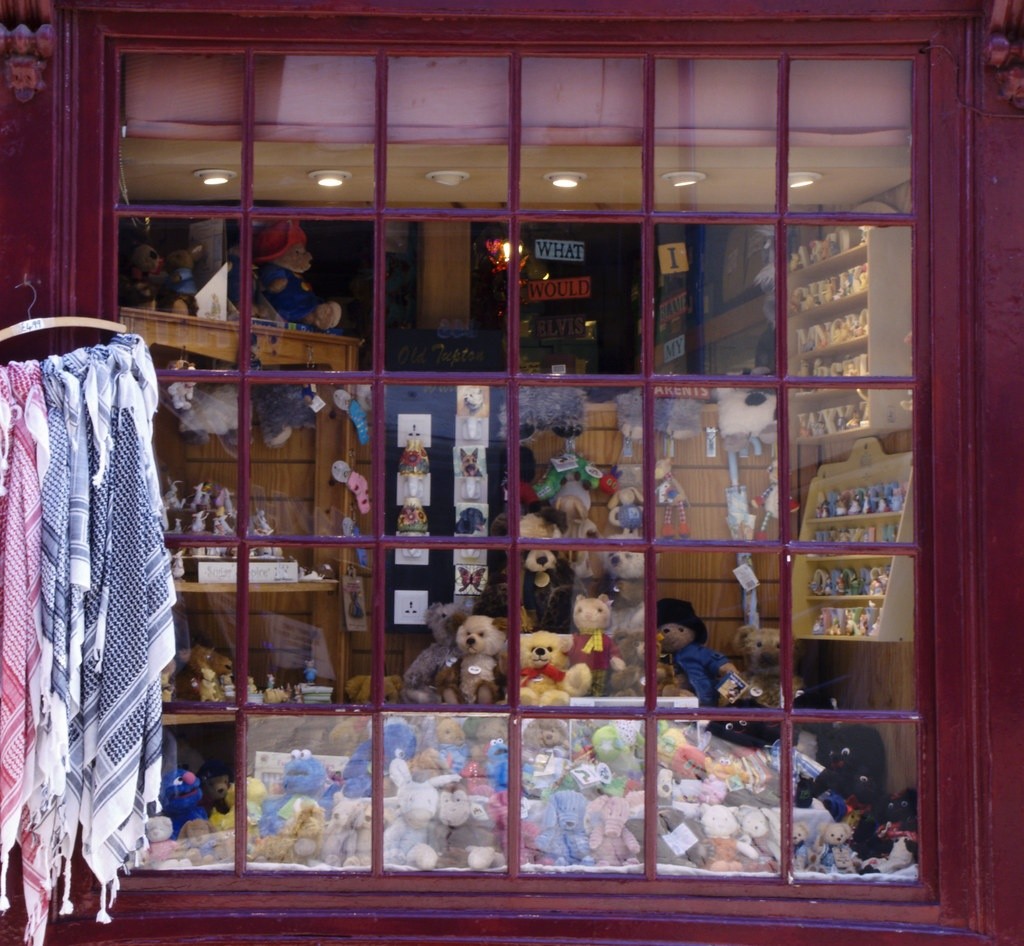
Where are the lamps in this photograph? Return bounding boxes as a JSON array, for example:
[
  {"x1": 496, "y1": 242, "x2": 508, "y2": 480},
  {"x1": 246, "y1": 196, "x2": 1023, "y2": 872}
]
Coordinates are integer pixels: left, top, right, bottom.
[
  {"x1": 787, "y1": 172, "x2": 821, "y2": 188},
  {"x1": 195, "y1": 169, "x2": 237, "y2": 185},
  {"x1": 662, "y1": 172, "x2": 706, "y2": 187},
  {"x1": 309, "y1": 170, "x2": 350, "y2": 187},
  {"x1": 544, "y1": 172, "x2": 586, "y2": 188},
  {"x1": 425, "y1": 171, "x2": 470, "y2": 186}
]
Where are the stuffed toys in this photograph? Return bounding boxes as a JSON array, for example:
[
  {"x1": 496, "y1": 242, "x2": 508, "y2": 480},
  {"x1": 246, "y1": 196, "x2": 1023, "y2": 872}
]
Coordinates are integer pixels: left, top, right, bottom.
[{"x1": 122, "y1": 217, "x2": 919, "y2": 889}]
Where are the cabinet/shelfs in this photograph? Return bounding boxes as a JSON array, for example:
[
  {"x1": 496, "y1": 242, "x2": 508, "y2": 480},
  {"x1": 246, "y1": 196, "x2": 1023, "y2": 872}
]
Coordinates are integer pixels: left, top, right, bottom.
[
  {"x1": 787, "y1": 226, "x2": 911, "y2": 443},
  {"x1": 795, "y1": 438, "x2": 917, "y2": 643},
  {"x1": 115, "y1": 308, "x2": 363, "y2": 863}
]
